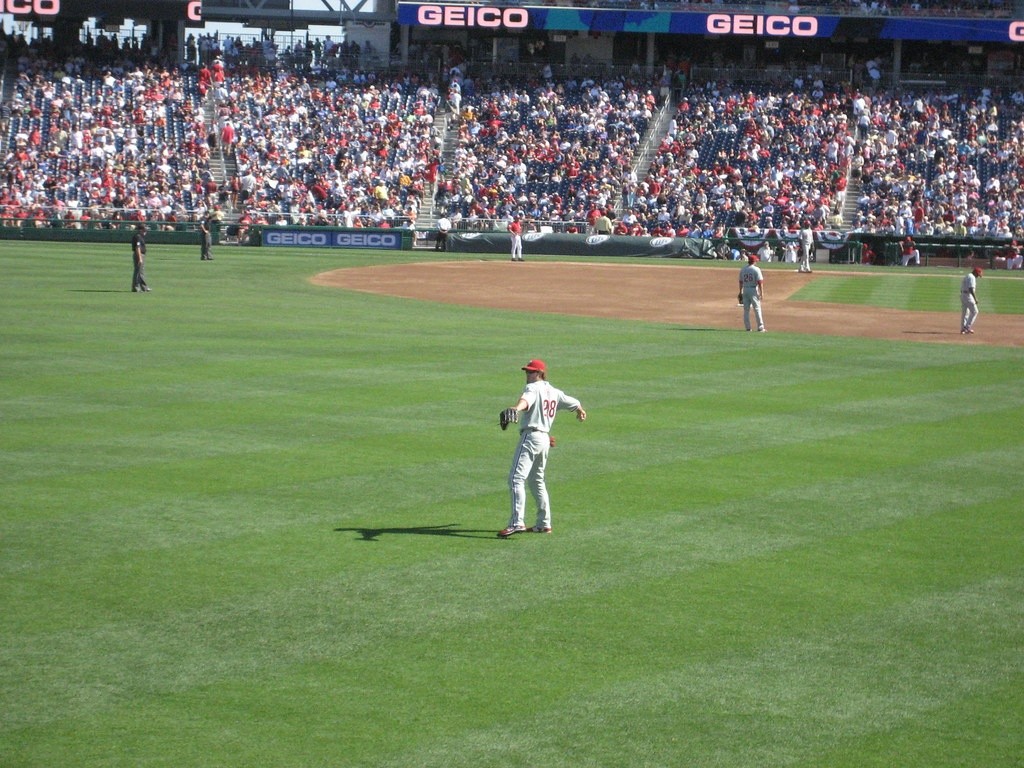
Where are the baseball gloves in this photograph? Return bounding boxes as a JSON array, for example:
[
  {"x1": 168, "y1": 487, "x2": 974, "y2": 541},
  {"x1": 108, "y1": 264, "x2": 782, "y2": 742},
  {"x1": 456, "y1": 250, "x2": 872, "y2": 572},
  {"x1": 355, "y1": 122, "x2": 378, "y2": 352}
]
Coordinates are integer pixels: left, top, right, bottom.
[
  {"x1": 497, "y1": 407, "x2": 521, "y2": 432},
  {"x1": 738, "y1": 293, "x2": 744, "y2": 306}
]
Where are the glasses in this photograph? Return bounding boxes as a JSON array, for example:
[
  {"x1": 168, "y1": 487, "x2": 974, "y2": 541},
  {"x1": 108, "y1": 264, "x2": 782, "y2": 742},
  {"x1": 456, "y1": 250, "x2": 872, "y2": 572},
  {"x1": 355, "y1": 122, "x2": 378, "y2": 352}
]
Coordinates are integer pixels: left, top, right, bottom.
[{"x1": 526, "y1": 371, "x2": 535, "y2": 374}]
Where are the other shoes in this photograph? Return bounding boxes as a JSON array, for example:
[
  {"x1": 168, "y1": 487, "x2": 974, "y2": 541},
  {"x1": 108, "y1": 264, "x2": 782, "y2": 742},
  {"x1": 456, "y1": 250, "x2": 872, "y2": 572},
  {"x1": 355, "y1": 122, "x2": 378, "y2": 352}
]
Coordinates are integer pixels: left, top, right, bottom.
[
  {"x1": 519, "y1": 258, "x2": 525, "y2": 261},
  {"x1": 144, "y1": 288, "x2": 151, "y2": 290},
  {"x1": 961, "y1": 326, "x2": 974, "y2": 334},
  {"x1": 414, "y1": 242, "x2": 416, "y2": 247},
  {"x1": 747, "y1": 328, "x2": 752, "y2": 331},
  {"x1": 512, "y1": 258, "x2": 516, "y2": 261},
  {"x1": 759, "y1": 329, "x2": 767, "y2": 332}
]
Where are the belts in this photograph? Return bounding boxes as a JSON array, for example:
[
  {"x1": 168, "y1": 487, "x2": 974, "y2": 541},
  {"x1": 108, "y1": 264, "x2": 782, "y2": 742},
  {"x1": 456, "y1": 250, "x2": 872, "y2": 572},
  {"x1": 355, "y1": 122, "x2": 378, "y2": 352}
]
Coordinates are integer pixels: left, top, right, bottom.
[
  {"x1": 961, "y1": 291, "x2": 968, "y2": 293},
  {"x1": 521, "y1": 428, "x2": 538, "y2": 432}
]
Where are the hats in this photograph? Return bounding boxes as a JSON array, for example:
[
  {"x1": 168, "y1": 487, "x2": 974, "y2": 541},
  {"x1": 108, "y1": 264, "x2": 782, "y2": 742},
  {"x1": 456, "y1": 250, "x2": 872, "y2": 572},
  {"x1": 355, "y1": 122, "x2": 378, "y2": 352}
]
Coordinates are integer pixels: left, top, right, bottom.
[
  {"x1": 522, "y1": 359, "x2": 546, "y2": 373},
  {"x1": 139, "y1": 224, "x2": 147, "y2": 229},
  {"x1": 514, "y1": 215, "x2": 520, "y2": 218},
  {"x1": 975, "y1": 267, "x2": 982, "y2": 273},
  {"x1": 601, "y1": 211, "x2": 606, "y2": 215},
  {"x1": 245, "y1": 211, "x2": 250, "y2": 214},
  {"x1": 317, "y1": 205, "x2": 322, "y2": 208},
  {"x1": 805, "y1": 220, "x2": 810, "y2": 225},
  {"x1": 749, "y1": 255, "x2": 759, "y2": 262},
  {"x1": 864, "y1": 244, "x2": 867, "y2": 248},
  {"x1": 907, "y1": 237, "x2": 910, "y2": 240},
  {"x1": 139, "y1": 203, "x2": 144, "y2": 207},
  {"x1": 137, "y1": 211, "x2": 141, "y2": 215},
  {"x1": 590, "y1": 203, "x2": 594, "y2": 207},
  {"x1": 214, "y1": 205, "x2": 219, "y2": 208}
]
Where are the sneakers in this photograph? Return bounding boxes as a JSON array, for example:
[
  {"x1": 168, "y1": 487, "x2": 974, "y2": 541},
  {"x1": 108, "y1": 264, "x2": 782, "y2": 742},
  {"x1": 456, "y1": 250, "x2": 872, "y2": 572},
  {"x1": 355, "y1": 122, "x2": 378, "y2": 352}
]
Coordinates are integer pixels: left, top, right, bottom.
[
  {"x1": 527, "y1": 526, "x2": 552, "y2": 534},
  {"x1": 500, "y1": 526, "x2": 526, "y2": 535}
]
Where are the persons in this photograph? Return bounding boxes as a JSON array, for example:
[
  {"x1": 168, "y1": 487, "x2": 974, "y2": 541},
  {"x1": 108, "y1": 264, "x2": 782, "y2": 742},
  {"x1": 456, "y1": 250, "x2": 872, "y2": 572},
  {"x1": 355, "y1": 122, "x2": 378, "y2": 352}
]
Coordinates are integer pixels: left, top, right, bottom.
[
  {"x1": 960, "y1": 266, "x2": 983, "y2": 335},
  {"x1": 494, "y1": 359, "x2": 586, "y2": 538},
  {"x1": 131, "y1": 223, "x2": 152, "y2": 292},
  {"x1": 0, "y1": 1, "x2": 1024, "y2": 271},
  {"x1": 738, "y1": 254, "x2": 769, "y2": 333}
]
